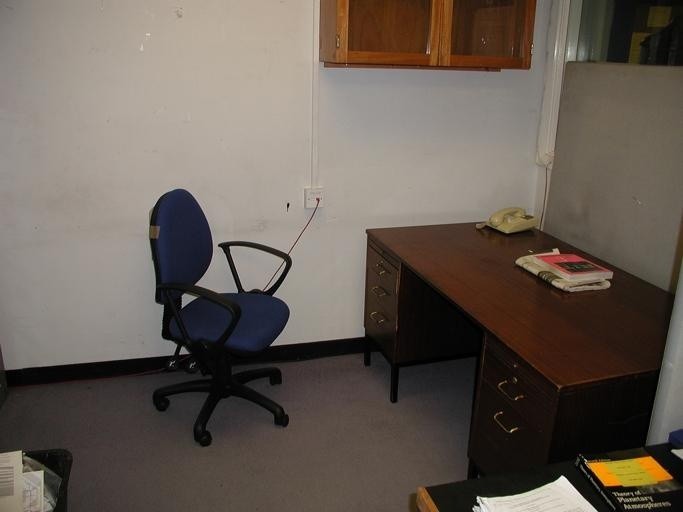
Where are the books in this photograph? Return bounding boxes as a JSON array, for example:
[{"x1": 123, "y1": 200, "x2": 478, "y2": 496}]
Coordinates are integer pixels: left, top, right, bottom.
[
  {"x1": 516, "y1": 247, "x2": 614, "y2": 294},
  {"x1": 0, "y1": 450, "x2": 45, "y2": 512},
  {"x1": 472, "y1": 429, "x2": 683, "y2": 512}
]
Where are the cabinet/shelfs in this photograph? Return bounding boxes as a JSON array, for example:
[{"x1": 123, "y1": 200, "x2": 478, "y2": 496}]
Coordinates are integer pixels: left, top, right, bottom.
[{"x1": 320, "y1": 0, "x2": 537, "y2": 73}]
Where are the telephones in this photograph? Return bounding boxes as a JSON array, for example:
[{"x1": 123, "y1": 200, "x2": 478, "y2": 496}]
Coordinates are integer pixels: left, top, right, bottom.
[{"x1": 485, "y1": 207, "x2": 538, "y2": 234}]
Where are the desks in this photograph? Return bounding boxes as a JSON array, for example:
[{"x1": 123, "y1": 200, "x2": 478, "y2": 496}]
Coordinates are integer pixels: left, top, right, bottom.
[
  {"x1": 364, "y1": 221, "x2": 675, "y2": 479},
  {"x1": 416, "y1": 442, "x2": 683, "y2": 512}
]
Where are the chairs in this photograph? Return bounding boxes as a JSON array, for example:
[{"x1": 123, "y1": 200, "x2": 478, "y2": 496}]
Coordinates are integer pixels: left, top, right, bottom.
[{"x1": 149, "y1": 189, "x2": 292, "y2": 447}]
